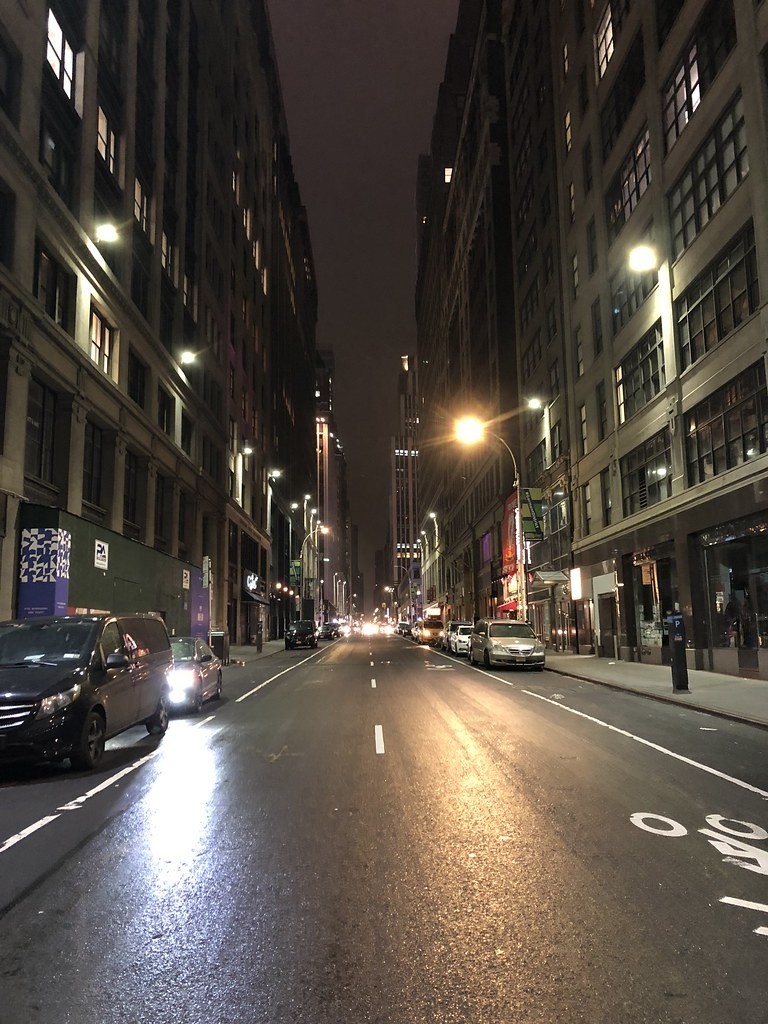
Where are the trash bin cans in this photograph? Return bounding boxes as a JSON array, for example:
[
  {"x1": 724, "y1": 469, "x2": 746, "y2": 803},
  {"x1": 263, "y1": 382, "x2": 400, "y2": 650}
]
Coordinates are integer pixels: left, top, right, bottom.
[{"x1": 207, "y1": 631, "x2": 229, "y2": 660}]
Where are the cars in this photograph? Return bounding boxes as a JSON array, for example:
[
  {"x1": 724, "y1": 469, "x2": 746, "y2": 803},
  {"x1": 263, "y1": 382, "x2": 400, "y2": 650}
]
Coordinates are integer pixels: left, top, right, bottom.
[
  {"x1": 468, "y1": 618, "x2": 546, "y2": 672},
  {"x1": 283, "y1": 620, "x2": 318, "y2": 650},
  {"x1": 0, "y1": 613, "x2": 175, "y2": 772},
  {"x1": 396, "y1": 619, "x2": 473, "y2": 658},
  {"x1": 169, "y1": 636, "x2": 223, "y2": 714},
  {"x1": 316, "y1": 622, "x2": 350, "y2": 641}
]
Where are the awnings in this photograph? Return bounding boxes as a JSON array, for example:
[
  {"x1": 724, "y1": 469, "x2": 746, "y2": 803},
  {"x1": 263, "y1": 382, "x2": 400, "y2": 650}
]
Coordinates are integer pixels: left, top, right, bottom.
[{"x1": 243, "y1": 589, "x2": 270, "y2": 606}]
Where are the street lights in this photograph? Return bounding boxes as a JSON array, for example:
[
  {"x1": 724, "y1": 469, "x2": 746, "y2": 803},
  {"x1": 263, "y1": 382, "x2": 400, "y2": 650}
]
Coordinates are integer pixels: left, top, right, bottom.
[
  {"x1": 300, "y1": 527, "x2": 329, "y2": 620},
  {"x1": 454, "y1": 416, "x2": 525, "y2": 623},
  {"x1": 393, "y1": 565, "x2": 413, "y2": 624},
  {"x1": 345, "y1": 593, "x2": 356, "y2": 616}
]
